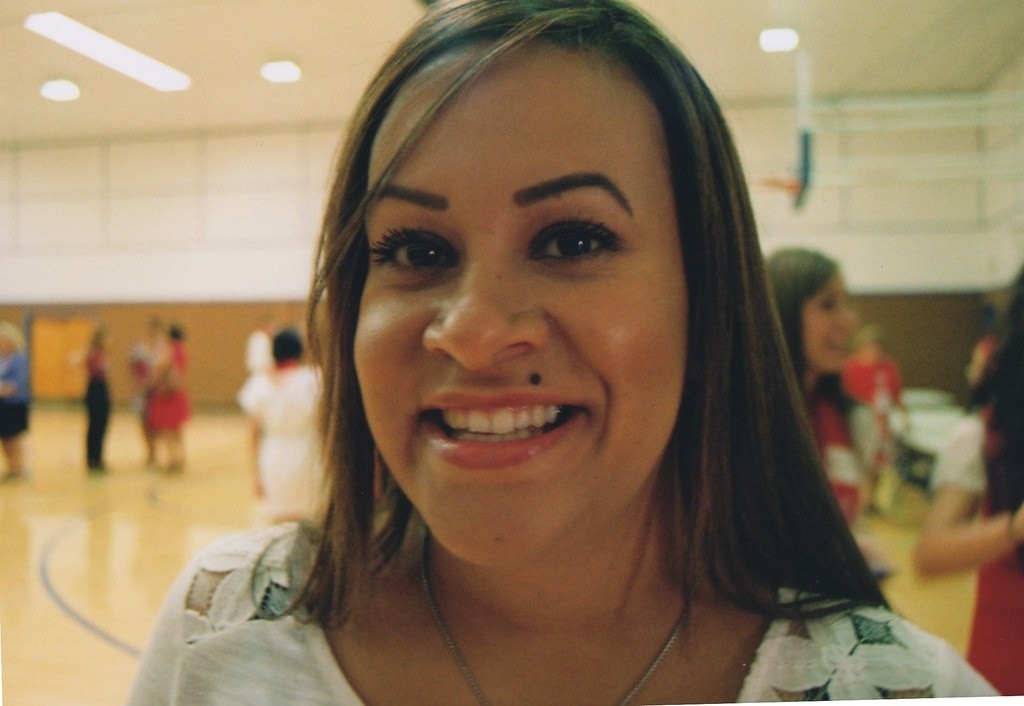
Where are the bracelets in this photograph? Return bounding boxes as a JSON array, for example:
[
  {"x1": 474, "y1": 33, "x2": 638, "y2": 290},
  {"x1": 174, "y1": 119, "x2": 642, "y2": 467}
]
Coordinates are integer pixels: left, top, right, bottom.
[{"x1": 1007, "y1": 504, "x2": 1022, "y2": 551}]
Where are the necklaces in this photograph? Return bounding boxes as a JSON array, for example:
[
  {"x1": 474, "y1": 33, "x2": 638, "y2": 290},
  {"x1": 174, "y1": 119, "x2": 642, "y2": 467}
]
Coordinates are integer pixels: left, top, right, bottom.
[{"x1": 419, "y1": 521, "x2": 691, "y2": 706}]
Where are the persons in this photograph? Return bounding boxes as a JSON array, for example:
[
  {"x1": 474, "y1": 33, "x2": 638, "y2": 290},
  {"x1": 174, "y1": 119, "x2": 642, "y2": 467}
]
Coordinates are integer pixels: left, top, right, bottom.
[
  {"x1": 123, "y1": 0, "x2": 1003, "y2": 706},
  {"x1": 0, "y1": 0, "x2": 1024, "y2": 706}
]
